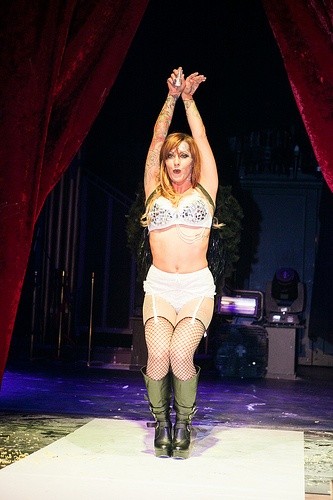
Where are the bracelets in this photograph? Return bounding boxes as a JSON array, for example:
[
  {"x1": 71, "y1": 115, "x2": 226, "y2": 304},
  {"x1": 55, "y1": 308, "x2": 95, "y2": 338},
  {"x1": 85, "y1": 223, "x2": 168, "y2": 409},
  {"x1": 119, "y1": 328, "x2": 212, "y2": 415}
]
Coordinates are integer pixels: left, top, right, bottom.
[{"x1": 182, "y1": 99, "x2": 194, "y2": 101}]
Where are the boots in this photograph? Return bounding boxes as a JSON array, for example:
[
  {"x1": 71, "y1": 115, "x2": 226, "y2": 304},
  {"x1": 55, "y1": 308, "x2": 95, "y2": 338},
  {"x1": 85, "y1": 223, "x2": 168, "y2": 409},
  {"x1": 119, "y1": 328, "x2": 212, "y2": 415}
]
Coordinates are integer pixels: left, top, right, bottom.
[
  {"x1": 173, "y1": 365, "x2": 201, "y2": 458},
  {"x1": 140, "y1": 366, "x2": 172, "y2": 457}
]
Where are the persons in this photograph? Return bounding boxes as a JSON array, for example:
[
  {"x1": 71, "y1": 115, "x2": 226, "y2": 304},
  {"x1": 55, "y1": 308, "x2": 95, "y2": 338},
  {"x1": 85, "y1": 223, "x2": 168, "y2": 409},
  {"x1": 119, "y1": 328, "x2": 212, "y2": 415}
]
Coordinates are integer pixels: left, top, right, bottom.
[{"x1": 140, "y1": 66, "x2": 219, "y2": 458}]
